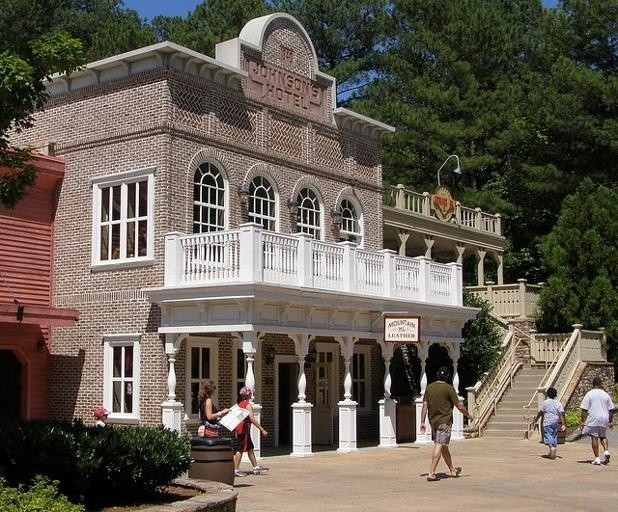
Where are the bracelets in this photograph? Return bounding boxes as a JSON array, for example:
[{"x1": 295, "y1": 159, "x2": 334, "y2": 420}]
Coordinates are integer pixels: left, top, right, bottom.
[{"x1": 216, "y1": 410, "x2": 222, "y2": 417}]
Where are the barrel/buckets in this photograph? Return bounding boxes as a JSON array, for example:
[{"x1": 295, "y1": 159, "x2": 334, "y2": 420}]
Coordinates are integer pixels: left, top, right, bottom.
[{"x1": 190, "y1": 435, "x2": 235, "y2": 486}]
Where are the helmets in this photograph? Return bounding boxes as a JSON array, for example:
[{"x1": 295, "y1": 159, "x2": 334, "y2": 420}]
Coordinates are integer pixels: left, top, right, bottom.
[{"x1": 436, "y1": 366, "x2": 450, "y2": 378}]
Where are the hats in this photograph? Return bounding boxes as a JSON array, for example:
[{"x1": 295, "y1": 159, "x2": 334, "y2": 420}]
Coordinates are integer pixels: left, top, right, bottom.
[
  {"x1": 94, "y1": 407, "x2": 107, "y2": 417},
  {"x1": 239, "y1": 387, "x2": 255, "y2": 398}
]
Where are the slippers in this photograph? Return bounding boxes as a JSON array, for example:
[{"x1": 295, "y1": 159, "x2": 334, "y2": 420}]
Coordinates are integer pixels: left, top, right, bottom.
[
  {"x1": 452, "y1": 467, "x2": 461, "y2": 478},
  {"x1": 427, "y1": 475, "x2": 440, "y2": 481}
]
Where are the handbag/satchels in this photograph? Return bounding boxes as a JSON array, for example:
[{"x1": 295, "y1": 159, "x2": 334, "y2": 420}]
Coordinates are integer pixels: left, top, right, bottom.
[{"x1": 205, "y1": 423, "x2": 219, "y2": 438}]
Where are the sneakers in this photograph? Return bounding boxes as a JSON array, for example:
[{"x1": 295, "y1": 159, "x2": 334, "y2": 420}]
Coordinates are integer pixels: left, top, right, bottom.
[
  {"x1": 549, "y1": 447, "x2": 556, "y2": 459},
  {"x1": 234, "y1": 469, "x2": 247, "y2": 477},
  {"x1": 252, "y1": 464, "x2": 269, "y2": 473},
  {"x1": 592, "y1": 459, "x2": 601, "y2": 465},
  {"x1": 601, "y1": 450, "x2": 611, "y2": 464}
]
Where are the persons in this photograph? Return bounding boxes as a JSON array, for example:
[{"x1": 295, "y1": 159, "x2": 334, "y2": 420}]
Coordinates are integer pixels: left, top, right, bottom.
[
  {"x1": 580, "y1": 376, "x2": 615, "y2": 465},
  {"x1": 420, "y1": 365, "x2": 476, "y2": 481},
  {"x1": 532, "y1": 386, "x2": 567, "y2": 460},
  {"x1": 233, "y1": 385, "x2": 270, "y2": 477},
  {"x1": 94, "y1": 406, "x2": 112, "y2": 427},
  {"x1": 198, "y1": 379, "x2": 231, "y2": 438}
]
